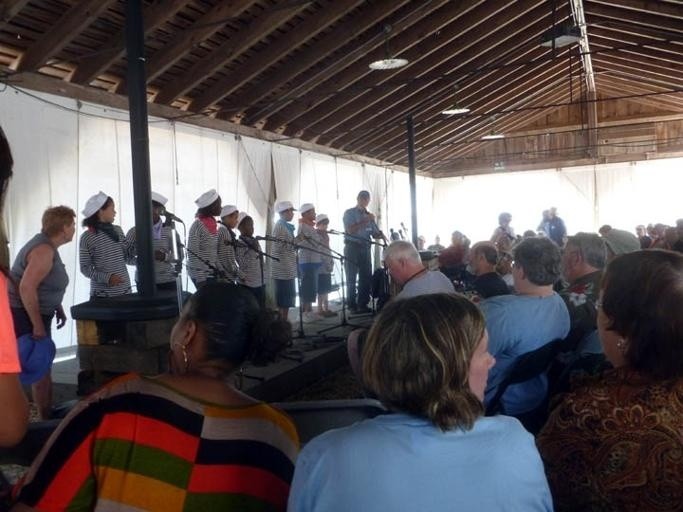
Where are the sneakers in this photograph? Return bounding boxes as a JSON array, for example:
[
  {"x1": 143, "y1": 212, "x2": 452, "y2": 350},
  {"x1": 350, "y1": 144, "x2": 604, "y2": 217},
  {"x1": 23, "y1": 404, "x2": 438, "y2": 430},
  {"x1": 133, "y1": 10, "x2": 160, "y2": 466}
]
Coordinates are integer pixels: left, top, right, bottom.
[
  {"x1": 319, "y1": 312, "x2": 329, "y2": 319},
  {"x1": 296, "y1": 311, "x2": 311, "y2": 324},
  {"x1": 307, "y1": 311, "x2": 319, "y2": 321},
  {"x1": 326, "y1": 310, "x2": 337, "y2": 318},
  {"x1": 349, "y1": 306, "x2": 359, "y2": 315},
  {"x1": 44, "y1": 404, "x2": 74, "y2": 418},
  {"x1": 359, "y1": 305, "x2": 369, "y2": 313}
]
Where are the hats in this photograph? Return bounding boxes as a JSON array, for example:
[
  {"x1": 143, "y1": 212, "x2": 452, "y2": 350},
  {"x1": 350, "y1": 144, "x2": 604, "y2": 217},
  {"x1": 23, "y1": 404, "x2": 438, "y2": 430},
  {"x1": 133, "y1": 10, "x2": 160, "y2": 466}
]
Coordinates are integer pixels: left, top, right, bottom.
[
  {"x1": 151, "y1": 190, "x2": 169, "y2": 207},
  {"x1": 299, "y1": 203, "x2": 314, "y2": 214},
  {"x1": 220, "y1": 204, "x2": 237, "y2": 217},
  {"x1": 275, "y1": 201, "x2": 298, "y2": 214},
  {"x1": 358, "y1": 190, "x2": 371, "y2": 199},
  {"x1": 194, "y1": 188, "x2": 219, "y2": 208},
  {"x1": 234, "y1": 212, "x2": 248, "y2": 228},
  {"x1": 315, "y1": 213, "x2": 327, "y2": 223},
  {"x1": 81, "y1": 191, "x2": 108, "y2": 218}
]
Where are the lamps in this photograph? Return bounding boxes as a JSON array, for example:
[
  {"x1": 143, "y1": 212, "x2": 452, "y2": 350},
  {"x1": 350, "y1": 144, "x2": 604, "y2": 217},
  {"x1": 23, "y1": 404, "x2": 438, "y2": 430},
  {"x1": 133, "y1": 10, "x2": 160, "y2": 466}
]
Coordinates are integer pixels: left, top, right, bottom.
[
  {"x1": 480, "y1": 117, "x2": 505, "y2": 140},
  {"x1": 368, "y1": 23, "x2": 409, "y2": 70},
  {"x1": 442, "y1": 86, "x2": 470, "y2": 115}
]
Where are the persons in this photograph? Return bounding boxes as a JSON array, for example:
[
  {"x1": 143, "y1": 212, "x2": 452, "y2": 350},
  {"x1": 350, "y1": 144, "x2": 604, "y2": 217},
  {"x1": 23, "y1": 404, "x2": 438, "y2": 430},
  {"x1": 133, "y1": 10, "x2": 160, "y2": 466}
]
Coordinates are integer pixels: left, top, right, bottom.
[
  {"x1": 654, "y1": 224, "x2": 666, "y2": 247},
  {"x1": 342, "y1": 190, "x2": 387, "y2": 315},
  {"x1": 557, "y1": 231, "x2": 611, "y2": 379},
  {"x1": 646, "y1": 224, "x2": 656, "y2": 246},
  {"x1": 490, "y1": 212, "x2": 516, "y2": 255},
  {"x1": 8, "y1": 204, "x2": 76, "y2": 423},
  {"x1": 235, "y1": 212, "x2": 266, "y2": 310},
  {"x1": 217, "y1": 204, "x2": 246, "y2": 284},
  {"x1": 270, "y1": 201, "x2": 304, "y2": 317},
  {"x1": 315, "y1": 213, "x2": 338, "y2": 318},
  {"x1": 536, "y1": 210, "x2": 548, "y2": 234},
  {"x1": 636, "y1": 224, "x2": 651, "y2": 248},
  {"x1": 548, "y1": 207, "x2": 567, "y2": 247},
  {"x1": 535, "y1": 247, "x2": 683, "y2": 512},
  {"x1": 126, "y1": 192, "x2": 184, "y2": 291},
  {"x1": 439, "y1": 231, "x2": 468, "y2": 284},
  {"x1": 286, "y1": 292, "x2": 553, "y2": 511},
  {"x1": 384, "y1": 240, "x2": 455, "y2": 301},
  {"x1": 186, "y1": 188, "x2": 225, "y2": 290},
  {"x1": 7, "y1": 281, "x2": 301, "y2": 512},
  {"x1": 297, "y1": 203, "x2": 323, "y2": 312},
  {"x1": 0, "y1": 125, "x2": 32, "y2": 453},
  {"x1": 481, "y1": 236, "x2": 571, "y2": 418},
  {"x1": 664, "y1": 227, "x2": 683, "y2": 251},
  {"x1": 599, "y1": 225, "x2": 642, "y2": 255},
  {"x1": 76, "y1": 191, "x2": 139, "y2": 301},
  {"x1": 466, "y1": 241, "x2": 511, "y2": 298}
]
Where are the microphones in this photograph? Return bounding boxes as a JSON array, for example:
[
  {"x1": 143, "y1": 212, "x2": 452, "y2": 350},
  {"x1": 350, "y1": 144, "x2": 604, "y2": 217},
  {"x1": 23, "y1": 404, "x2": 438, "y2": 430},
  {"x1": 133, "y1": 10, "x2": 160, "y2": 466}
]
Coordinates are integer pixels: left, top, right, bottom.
[
  {"x1": 157, "y1": 207, "x2": 182, "y2": 223},
  {"x1": 391, "y1": 229, "x2": 395, "y2": 241},
  {"x1": 326, "y1": 231, "x2": 338, "y2": 234},
  {"x1": 256, "y1": 236, "x2": 275, "y2": 241},
  {"x1": 400, "y1": 222, "x2": 409, "y2": 240},
  {"x1": 301, "y1": 232, "x2": 315, "y2": 248},
  {"x1": 399, "y1": 230, "x2": 404, "y2": 239},
  {"x1": 224, "y1": 240, "x2": 243, "y2": 248}
]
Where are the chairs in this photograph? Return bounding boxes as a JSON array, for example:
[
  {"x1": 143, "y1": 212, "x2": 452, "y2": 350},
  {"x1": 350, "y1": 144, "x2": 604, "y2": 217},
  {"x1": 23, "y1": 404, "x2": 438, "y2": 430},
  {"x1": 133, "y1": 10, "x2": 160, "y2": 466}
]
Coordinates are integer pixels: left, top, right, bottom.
[{"x1": 272, "y1": 398, "x2": 386, "y2": 441}]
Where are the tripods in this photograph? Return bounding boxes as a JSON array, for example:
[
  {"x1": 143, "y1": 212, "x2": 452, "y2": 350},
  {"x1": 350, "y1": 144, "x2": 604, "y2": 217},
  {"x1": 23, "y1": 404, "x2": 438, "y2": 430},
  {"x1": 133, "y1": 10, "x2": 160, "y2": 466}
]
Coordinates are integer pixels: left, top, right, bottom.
[
  {"x1": 234, "y1": 242, "x2": 302, "y2": 365},
  {"x1": 330, "y1": 229, "x2": 387, "y2": 319},
  {"x1": 266, "y1": 235, "x2": 341, "y2": 340},
  {"x1": 304, "y1": 236, "x2": 368, "y2": 334},
  {"x1": 176, "y1": 240, "x2": 267, "y2": 391}
]
